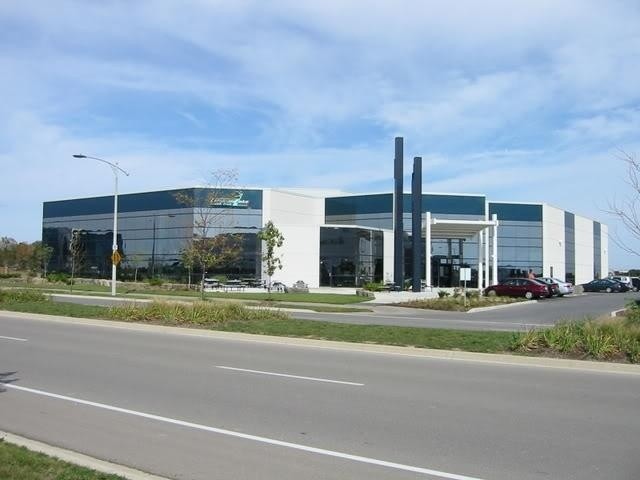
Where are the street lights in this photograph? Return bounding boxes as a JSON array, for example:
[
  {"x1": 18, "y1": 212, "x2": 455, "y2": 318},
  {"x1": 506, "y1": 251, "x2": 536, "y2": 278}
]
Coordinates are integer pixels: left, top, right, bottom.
[{"x1": 73, "y1": 154, "x2": 130, "y2": 297}]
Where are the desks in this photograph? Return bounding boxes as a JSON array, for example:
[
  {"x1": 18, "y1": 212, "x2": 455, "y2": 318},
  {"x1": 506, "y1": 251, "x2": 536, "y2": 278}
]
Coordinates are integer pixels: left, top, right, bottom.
[
  {"x1": 386, "y1": 282, "x2": 396, "y2": 292},
  {"x1": 242, "y1": 279, "x2": 266, "y2": 288},
  {"x1": 227, "y1": 280, "x2": 241, "y2": 290},
  {"x1": 205, "y1": 278, "x2": 219, "y2": 290}
]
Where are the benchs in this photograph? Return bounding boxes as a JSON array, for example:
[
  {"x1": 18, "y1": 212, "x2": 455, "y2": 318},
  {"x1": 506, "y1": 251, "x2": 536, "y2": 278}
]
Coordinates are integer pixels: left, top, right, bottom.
[
  {"x1": 384, "y1": 286, "x2": 401, "y2": 294},
  {"x1": 203, "y1": 283, "x2": 246, "y2": 292},
  {"x1": 239, "y1": 282, "x2": 268, "y2": 288}
]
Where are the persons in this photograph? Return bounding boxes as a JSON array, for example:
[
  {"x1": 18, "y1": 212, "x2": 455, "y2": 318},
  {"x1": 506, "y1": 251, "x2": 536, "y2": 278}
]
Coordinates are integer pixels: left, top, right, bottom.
[{"x1": 528, "y1": 268, "x2": 537, "y2": 282}]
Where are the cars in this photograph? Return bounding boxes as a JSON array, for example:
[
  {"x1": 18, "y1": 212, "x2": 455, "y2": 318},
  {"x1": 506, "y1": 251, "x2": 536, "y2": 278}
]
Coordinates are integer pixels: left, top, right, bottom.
[
  {"x1": 482, "y1": 277, "x2": 574, "y2": 300},
  {"x1": 581, "y1": 275, "x2": 640, "y2": 293}
]
